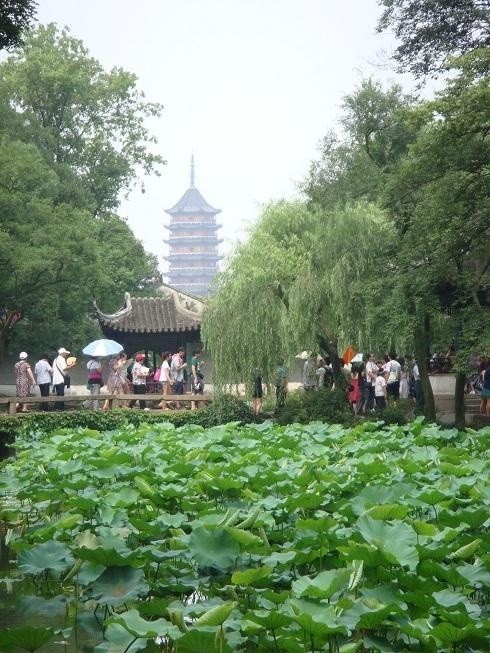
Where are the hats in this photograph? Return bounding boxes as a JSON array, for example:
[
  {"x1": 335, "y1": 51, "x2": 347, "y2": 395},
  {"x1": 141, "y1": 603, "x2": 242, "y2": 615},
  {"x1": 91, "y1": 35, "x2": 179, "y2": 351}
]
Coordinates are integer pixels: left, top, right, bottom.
[
  {"x1": 19, "y1": 352, "x2": 28, "y2": 360},
  {"x1": 58, "y1": 348, "x2": 70, "y2": 354},
  {"x1": 136, "y1": 354, "x2": 145, "y2": 361}
]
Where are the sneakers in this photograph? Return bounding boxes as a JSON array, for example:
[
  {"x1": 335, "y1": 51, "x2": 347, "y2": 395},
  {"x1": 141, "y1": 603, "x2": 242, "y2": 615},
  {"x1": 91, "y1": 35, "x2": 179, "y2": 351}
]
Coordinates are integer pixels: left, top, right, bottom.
[{"x1": 22, "y1": 409, "x2": 30, "y2": 413}]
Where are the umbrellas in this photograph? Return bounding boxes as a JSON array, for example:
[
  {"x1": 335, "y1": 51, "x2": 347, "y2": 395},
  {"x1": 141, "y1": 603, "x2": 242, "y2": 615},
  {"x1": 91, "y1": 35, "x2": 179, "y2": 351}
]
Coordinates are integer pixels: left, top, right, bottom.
[{"x1": 81, "y1": 338, "x2": 124, "y2": 365}]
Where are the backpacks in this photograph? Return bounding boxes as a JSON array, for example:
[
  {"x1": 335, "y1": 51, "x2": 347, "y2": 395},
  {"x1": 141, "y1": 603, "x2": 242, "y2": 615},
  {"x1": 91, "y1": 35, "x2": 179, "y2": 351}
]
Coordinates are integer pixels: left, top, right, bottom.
[{"x1": 323, "y1": 367, "x2": 334, "y2": 387}]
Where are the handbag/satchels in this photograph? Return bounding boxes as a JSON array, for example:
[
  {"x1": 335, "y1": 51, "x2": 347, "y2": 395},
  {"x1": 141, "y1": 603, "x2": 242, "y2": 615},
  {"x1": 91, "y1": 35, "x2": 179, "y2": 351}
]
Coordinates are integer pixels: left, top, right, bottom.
[
  {"x1": 193, "y1": 382, "x2": 203, "y2": 392},
  {"x1": 154, "y1": 368, "x2": 160, "y2": 381},
  {"x1": 64, "y1": 376, "x2": 70, "y2": 385},
  {"x1": 348, "y1": 385, "x2": 354, "y2": 392},
  {"x1": 473, "y1": 376, "x2": 484, "y2": 390},
  {"x1": 87, "y1": 379, "x2": 104, "y2": 390}
]
